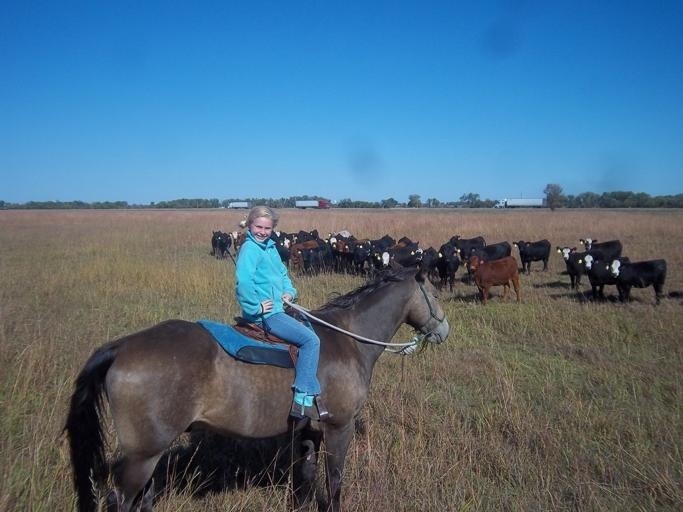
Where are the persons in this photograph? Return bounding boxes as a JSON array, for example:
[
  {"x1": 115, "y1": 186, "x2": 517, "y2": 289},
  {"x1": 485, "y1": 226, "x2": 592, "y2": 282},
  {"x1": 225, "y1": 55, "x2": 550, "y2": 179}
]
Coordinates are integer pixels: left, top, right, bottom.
[{"x1": 235, "y1": 205, "x2": 337, "y2": 424}]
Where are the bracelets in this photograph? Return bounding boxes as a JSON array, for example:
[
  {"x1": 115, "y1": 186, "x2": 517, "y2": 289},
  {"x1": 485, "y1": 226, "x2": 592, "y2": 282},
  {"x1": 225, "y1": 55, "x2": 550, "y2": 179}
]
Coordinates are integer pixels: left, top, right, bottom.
[{"x1": 259, "y1": 303, "x2": 265, "y2": 314}]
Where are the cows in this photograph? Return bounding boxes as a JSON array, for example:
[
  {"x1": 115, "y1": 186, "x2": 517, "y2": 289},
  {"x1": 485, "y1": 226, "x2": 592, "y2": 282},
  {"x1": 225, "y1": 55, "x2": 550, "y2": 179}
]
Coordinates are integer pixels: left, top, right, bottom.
[
  {"x1": 512, "y1": 239, "x2": 551, "y2": 276},
  {"x1": 211, "y1": 231, "x2": 522, "y2": 305},
  {"x1": 555, "y1": 237, "x2": 667, "y2": 306}
]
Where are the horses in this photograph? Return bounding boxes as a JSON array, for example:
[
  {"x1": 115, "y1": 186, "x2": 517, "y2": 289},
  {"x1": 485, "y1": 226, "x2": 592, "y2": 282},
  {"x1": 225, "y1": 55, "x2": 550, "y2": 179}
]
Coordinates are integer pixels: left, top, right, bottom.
[{"x1": 62, "y1": 266, "x2": 449, "y2": 512}]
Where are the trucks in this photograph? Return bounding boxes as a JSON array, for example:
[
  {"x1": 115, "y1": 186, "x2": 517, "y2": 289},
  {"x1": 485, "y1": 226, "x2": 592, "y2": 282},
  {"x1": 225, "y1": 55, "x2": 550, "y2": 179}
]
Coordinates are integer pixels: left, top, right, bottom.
[
  {"x1": 494, "y1": 198, "x2": 543, "y2": 208},
  {"x1": 295, "y1": 198, "x2": 330, "y2": 209},
  {"x1": 227, "y1": 201, "x2": 249, "y2": 209}
]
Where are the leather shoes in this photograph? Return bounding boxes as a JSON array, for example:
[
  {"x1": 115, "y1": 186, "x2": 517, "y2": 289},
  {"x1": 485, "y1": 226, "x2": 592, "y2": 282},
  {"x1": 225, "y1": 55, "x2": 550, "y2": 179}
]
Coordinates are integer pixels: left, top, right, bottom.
[{"x1": 290, "y1": 401, "x2": 333, "y2": 420}]
